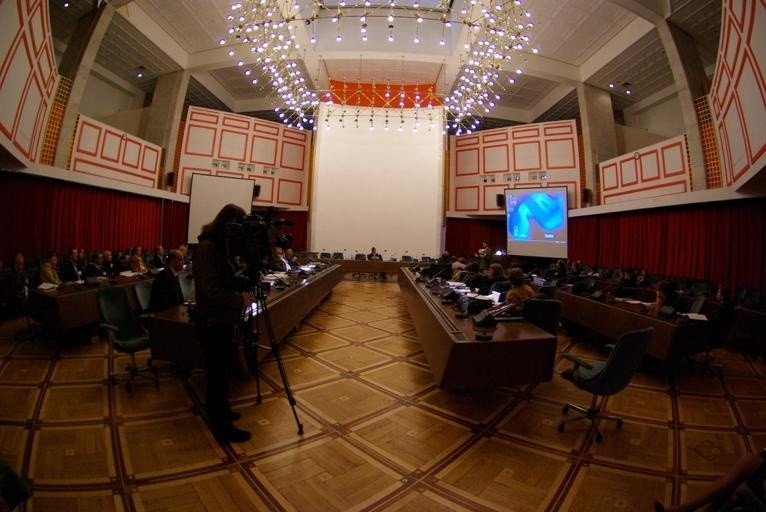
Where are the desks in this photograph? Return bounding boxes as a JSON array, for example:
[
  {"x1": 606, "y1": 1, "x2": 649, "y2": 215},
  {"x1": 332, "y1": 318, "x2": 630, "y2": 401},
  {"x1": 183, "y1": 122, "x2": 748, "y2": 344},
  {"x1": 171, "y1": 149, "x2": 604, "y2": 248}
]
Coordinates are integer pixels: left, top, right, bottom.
[
  {"x1": 32, "y1": 265, "x2": 152, "y2": 365},
  {"x1": 398, "y1": 264, "x2": 694, "y2": 397},
  {"x1": 301, "y1": 258, "x2": 430, "y2": 275},
  {"x1": 150, "y1": 263, "x2": 340, "y2": 374}
]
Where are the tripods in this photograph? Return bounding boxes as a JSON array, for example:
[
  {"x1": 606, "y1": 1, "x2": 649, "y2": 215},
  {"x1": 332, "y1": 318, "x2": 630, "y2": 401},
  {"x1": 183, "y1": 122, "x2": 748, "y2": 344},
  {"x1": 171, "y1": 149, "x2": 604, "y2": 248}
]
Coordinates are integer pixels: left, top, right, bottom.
[{"x1": 233, "y1": 273, "x2": 306, "y2": 434}]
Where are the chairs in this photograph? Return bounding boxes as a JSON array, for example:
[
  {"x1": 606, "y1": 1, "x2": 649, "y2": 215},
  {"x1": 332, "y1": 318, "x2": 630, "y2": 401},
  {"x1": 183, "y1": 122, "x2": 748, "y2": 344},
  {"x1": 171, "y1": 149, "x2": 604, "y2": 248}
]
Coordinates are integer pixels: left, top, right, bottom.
[
  {"x1": 98, "y1": 270, "x2": 192, "y2": 394},
  {"x1": 305, "y1": 252, "x2": 432, "y2": 261},
  {"x1": 558, "y1": 326, "x2": 654, "y2": 442},
  {"x1": 689, "y1": 296, "x2": 707, "y2": 320}
]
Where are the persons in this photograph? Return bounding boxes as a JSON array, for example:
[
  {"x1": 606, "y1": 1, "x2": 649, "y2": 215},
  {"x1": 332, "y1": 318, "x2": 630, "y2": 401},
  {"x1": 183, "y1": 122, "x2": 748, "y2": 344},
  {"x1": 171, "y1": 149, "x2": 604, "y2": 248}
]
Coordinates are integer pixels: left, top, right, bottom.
[
  {"x1": 438, "y1": 252, "x2": 649, "y2": 311},
  {"x1": 368, "y1": 247, "x2": 385, "y2": 279},
  {"x1": 656, "y1": 280, "x2": 679, "y2": 308},
  {"x1": 7, "y1": 245, "x2": 190, "y2": 315},
  {"x1": 194, "y1": 204, "x2": 258, "y2": 442},
  {"x1": 479, "y1": 240, "x2": 492, "y2": 262},
  {"x1": 701, "y1": 283, "x2": 738, "y2": 342},
  {"x1": 238, "y1": 246, "x2": 301, "y2": 276}
]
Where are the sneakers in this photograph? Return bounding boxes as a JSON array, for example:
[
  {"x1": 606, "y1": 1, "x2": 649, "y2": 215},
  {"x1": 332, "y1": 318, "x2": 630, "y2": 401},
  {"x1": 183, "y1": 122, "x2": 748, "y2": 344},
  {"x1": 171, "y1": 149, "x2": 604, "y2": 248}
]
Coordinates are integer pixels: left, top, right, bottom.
[{"x1": 213, "y1": 411, "x2": 251, "y2": 444}]
